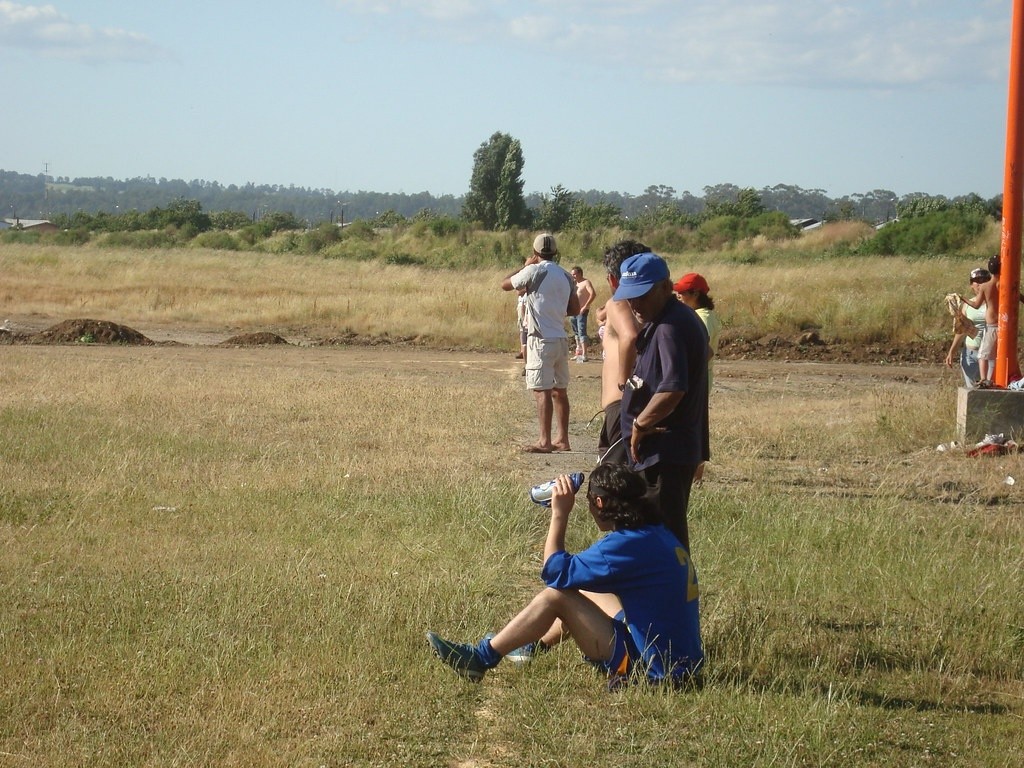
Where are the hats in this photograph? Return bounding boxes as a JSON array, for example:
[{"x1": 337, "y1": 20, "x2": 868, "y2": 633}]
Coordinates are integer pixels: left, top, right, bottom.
[
  {"x1": 988, "y1": 255, "x2": 1001, "y2": 267},
  {"x1": 970, "y1": 267, "x2": 991, "y2": 283},
  {"x1": 613, "y1": 253, "x2": 670, "y2": 301},
  {"x1": 672, "y1": 273, "x2": 709, "y2": 295},
  {"x1": 533, "y1": 234, "x2": 556, "y2": 254}
]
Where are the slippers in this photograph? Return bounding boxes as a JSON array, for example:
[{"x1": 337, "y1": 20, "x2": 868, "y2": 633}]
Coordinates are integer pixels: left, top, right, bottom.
[
  {"x1": 522, "y1": 445, "x2": 552, "y2": 453},
  {"x1": 551, "y1": 441, "x2": 571, "y2": 451}
]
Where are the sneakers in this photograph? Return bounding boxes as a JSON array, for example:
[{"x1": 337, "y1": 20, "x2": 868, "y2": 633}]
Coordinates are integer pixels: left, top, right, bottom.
[
  {"x1": 484, "y1": 632, "x2": 535, "y2": 663},
  {"x1": 976, "y1": 432, "x2": 1006, "y2": 447},
  {"x1": 427, "y1": 632, "x2": 487, "y2": 683}
]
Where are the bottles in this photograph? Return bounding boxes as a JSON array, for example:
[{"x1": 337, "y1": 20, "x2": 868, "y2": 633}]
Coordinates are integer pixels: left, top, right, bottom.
[{"x1": 576, "y1": 344, "x2": 583, "y2": 364}]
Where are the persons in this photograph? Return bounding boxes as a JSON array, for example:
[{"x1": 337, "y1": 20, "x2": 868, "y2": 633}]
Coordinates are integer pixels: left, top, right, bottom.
[
  {"x1": 502, "y1": 235, "x2": 716, "y2": 558},
  {"x1": 426, "y1": 461, "x2": 702, "y2": 688},
  {"x1": 944, "y1": 256, "x2": 1024, "y2": 389}
]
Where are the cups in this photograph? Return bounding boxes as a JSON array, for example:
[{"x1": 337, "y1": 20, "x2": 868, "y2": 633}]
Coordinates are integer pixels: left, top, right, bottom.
[{"x1": 530, "y1": 471, "x2": 584, "y2": 508}]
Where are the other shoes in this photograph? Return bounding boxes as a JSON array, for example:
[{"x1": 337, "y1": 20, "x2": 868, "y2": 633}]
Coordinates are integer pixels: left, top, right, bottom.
[
  {"x1": 515, "y1": 352, "x2": 523, "y2": 359},
  {"x1": 951, "y1": 441, "x2": 962, "y2": 449},
  {"x1": 522, "y1": 366, "x2": 526, "y2": 376},
  {"x1": 570, "y1": 354, "x2": 588, "y2": 362},
  {"x1": 937, "y1": 443, "x2": 950, "y2": 451},
  {"x1": 973, "y1": 379, "x2": 993, "y2": 389},
  {"x1": 1007, "y1": 381, "x2": 1024, "y2": 391}
]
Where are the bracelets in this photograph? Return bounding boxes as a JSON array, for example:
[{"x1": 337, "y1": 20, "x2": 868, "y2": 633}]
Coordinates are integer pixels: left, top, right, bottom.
[{"x1": 633, "y1": 417, "x2": 648, "y2": 432}]
